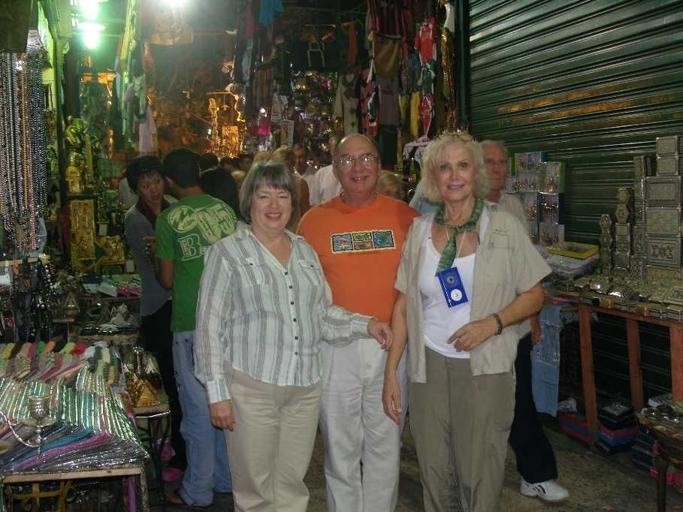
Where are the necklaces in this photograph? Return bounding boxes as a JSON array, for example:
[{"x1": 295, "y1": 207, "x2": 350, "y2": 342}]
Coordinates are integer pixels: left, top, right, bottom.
[{"x1": 1, "y1": 49, "x2": 50, "y2": 255}]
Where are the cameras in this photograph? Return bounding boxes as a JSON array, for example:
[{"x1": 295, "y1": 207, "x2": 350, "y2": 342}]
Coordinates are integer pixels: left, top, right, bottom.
[{"x1": 438, "y1": 267, "x2": 468, "y2": 308}]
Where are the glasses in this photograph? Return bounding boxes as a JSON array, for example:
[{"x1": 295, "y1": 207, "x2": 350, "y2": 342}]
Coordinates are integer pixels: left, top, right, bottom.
[{"x1": 331, "y1": 152, "x2": 380, "y2": 172}]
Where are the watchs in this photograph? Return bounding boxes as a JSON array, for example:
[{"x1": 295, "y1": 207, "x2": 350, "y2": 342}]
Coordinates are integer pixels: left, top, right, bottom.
[{"x1": 488, "y1": 312, "x2": 503, "y2": 337}]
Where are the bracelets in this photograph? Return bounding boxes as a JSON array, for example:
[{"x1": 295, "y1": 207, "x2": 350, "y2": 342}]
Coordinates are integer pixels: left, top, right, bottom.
[{"x1": 0, "y1": 257, "x2": 61, "y2": 345}]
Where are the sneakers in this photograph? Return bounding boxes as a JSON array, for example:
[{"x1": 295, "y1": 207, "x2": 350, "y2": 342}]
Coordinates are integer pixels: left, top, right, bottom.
[
  {"x1": 520, "y1": 477, "x2": 571, "y2": 503},
  {"x1": 160, "y1": 443, "x2": 173, "y2": 459},
  {"x1": 165, "y1": 488, "x2": 234, "y2": 511},
  {"x1": 160, "y1": 465, "x2": 185, "y2": 483}
]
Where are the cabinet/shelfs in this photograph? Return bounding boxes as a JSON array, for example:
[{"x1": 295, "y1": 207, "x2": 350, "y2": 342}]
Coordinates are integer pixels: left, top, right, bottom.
[{"x1": 537, "y1": 288, "x2": 682, "y2": 496}]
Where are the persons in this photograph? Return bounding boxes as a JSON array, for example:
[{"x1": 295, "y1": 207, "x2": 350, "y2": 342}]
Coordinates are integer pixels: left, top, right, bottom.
[
  {"x1": 155, "y1": 146, "x2": 240, "y2": 509},
  {"x1": 473, "y1": 137, "x2": 572, "y2": 505},
  {"x1": 192, "y1": 157, "x2": 395, "y2": 512},
  {"x1": 379, "y1": 130, "x2": 553, "y2": 511},
  {"x1": 295, "y1": 132, "x2": 423, "y2": 512}
]
